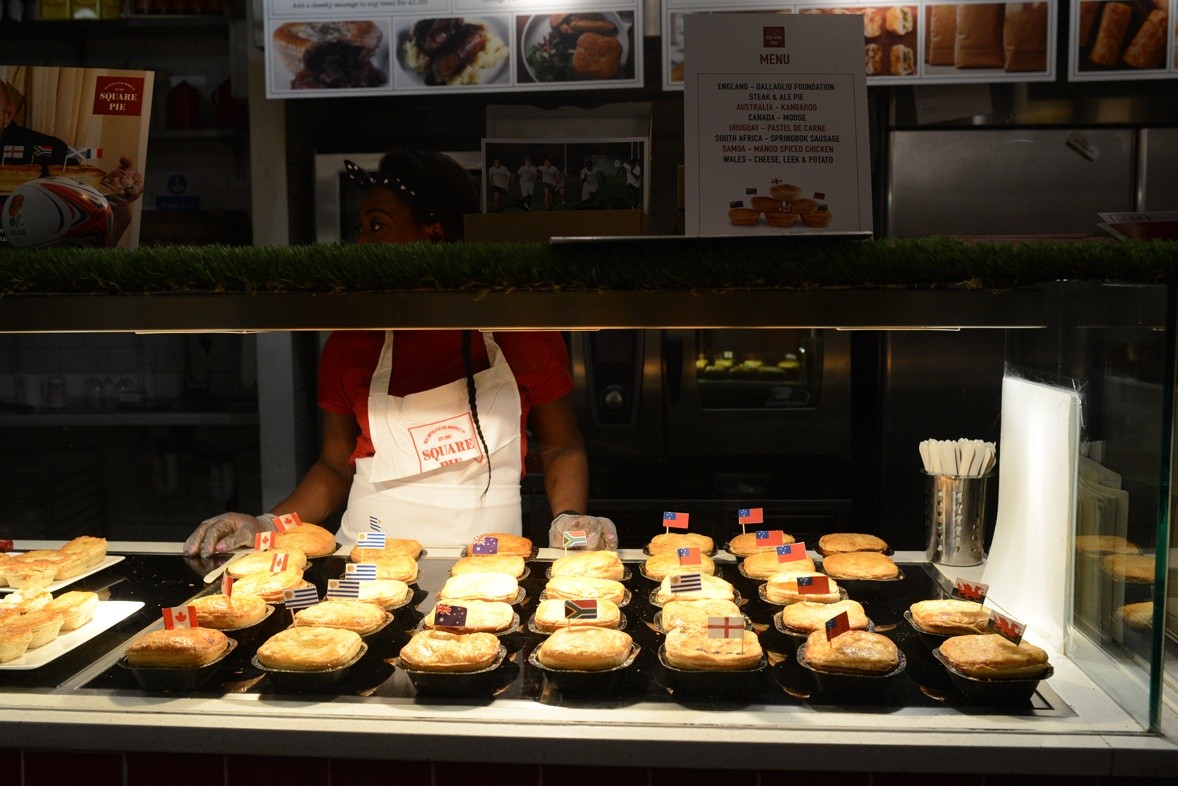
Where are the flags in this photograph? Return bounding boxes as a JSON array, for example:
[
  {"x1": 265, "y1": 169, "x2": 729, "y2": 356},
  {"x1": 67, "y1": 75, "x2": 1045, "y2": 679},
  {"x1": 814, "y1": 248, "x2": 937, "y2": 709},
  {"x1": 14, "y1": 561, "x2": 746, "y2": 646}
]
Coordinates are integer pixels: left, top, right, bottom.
[
  {"x1": 951, "y1": 576, "x2": 1027, "y2": 646},
  {"x1": 1, "y1": 145, "x2": 104, "y2": 171},
  {"x1": 161, "y1": 509, "x2": 851, "y2": 649}
]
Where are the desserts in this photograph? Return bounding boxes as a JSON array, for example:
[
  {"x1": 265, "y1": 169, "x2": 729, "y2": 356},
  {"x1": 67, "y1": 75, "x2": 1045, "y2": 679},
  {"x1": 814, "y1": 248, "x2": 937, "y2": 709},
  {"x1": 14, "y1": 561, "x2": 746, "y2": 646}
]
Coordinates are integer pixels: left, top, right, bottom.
[
  {"x1": 730, "y1": 186, "x2": 832, "y2": 227},
  {"x1": 0, "y1": 528, "x2": 1050, "y2": 705}
]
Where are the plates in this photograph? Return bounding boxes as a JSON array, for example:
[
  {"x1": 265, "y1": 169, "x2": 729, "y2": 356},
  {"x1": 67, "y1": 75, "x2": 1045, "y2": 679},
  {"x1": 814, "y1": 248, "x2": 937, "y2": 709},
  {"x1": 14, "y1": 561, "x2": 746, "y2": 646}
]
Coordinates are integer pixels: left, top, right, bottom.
[
  {"x1": 0, "y1": 601, "x2": 145, "y2": 669},
  {"x1": 519, "y1": 9, "x2": 629, "y2": 85},
  {"x1": 397, "y1": 17, "x2": 511, "y2": 87},
  {"x1": 0, "y1": 551, "x2": 128, "y2": 593}
]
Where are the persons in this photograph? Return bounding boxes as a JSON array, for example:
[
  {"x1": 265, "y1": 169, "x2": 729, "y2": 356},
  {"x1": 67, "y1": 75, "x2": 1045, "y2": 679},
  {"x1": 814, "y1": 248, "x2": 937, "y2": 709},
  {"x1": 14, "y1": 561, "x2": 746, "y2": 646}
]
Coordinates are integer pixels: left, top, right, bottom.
[
  {"x1": 179, "y1": 151, "x2": 619, "y2": 561},
  {"x1": 0, "y1": 79, "x2": 80, "y2": 165}
]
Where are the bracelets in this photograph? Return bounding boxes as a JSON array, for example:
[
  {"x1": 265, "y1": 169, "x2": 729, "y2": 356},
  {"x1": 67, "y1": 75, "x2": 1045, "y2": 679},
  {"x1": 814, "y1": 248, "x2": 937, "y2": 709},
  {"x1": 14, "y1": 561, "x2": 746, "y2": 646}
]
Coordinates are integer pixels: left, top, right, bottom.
[{"x1": 551, "y1": 510, "x2": 579, "y2": 517}]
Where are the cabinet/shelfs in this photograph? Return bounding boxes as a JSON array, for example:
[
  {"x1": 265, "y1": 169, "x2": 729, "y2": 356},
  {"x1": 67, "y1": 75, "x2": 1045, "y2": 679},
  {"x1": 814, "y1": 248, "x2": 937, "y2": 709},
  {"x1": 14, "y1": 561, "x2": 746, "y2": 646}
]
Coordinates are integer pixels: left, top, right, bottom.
[{"x1": 1, "y1": 0, "x2": 309, "y2": 526}]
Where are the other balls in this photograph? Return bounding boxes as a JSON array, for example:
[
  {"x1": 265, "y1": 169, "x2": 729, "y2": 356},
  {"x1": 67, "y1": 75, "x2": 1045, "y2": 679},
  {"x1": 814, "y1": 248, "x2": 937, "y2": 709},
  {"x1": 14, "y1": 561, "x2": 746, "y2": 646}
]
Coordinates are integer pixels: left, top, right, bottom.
[{"x1": 1, "y1": 175, "x2": 115, "y2": 250}]
